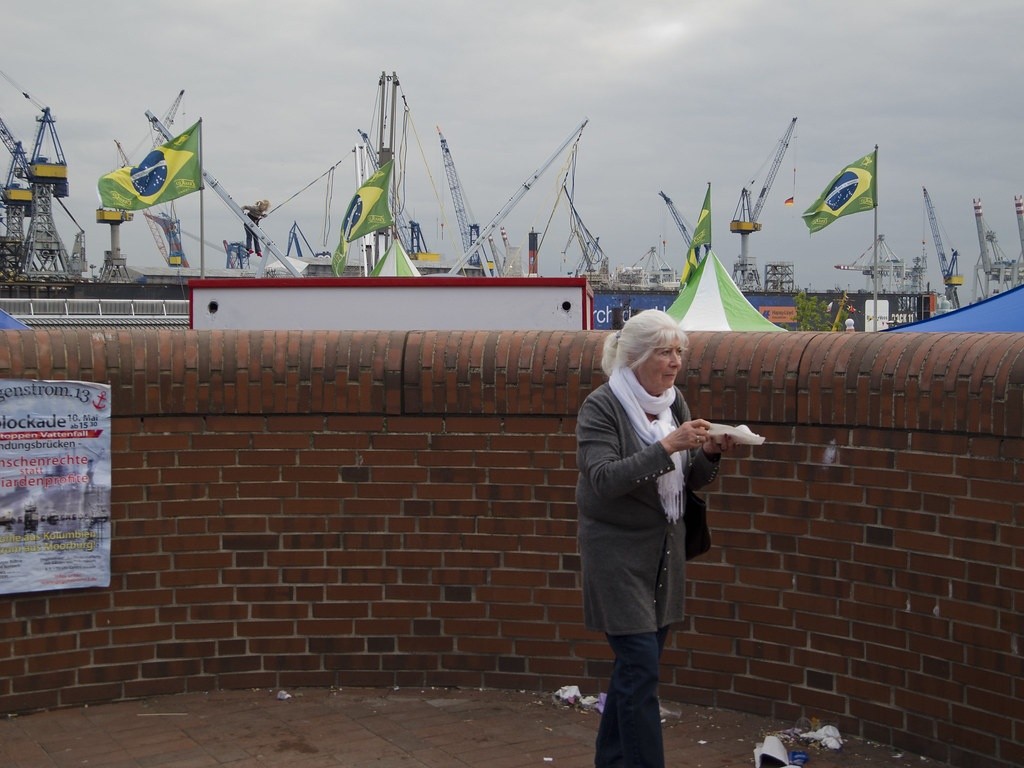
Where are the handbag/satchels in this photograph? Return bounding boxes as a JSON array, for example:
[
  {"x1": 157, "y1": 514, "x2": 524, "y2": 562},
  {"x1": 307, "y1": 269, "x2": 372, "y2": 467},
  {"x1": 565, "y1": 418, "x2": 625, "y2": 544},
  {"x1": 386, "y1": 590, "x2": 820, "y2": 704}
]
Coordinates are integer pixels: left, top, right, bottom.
[{"x1": 683, "y1": 487, "x2": 711, "y2": 561}]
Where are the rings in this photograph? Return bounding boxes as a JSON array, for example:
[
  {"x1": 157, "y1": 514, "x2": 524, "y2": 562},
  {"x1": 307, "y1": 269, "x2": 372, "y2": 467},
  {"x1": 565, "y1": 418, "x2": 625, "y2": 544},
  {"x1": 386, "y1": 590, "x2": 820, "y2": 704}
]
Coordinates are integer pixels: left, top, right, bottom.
[
  {"x1": 720, "y1": 447, "x2": 726, "y2": 452},
  {"x1": 696, "y1": 436, "x2": 700, "y2": 443}
]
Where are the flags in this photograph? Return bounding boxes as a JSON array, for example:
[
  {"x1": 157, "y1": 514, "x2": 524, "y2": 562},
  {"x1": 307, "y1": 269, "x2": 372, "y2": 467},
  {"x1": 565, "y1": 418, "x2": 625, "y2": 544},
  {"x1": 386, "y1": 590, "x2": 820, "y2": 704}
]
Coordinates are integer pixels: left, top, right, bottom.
[
  {"x1": 332, "y1": 159, "x2": 396, "y2": 277},
  {"x1": 98, "y1": 117, "x2": 205, "y2": 210},
  {"x1": 802, "y1": 149, "x2": 878, "y2": 233},
  {"x1": 681, "y1": 182, "x2": 712, "y2": 290},
  {"x1": 785, "y1": 197, "x2": 793, "y2": 206}
]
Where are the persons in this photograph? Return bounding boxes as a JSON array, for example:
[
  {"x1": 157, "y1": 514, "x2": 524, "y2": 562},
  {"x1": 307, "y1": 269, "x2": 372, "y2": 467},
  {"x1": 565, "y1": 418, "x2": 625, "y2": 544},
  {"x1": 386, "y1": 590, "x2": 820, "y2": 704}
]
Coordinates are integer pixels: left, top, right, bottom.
[
  {"x1": 241, "y1": 199, "x2": 270, "y2": 257},
  {"x1": 575, "y1": 309, "x2": 740, "y2": 768}
]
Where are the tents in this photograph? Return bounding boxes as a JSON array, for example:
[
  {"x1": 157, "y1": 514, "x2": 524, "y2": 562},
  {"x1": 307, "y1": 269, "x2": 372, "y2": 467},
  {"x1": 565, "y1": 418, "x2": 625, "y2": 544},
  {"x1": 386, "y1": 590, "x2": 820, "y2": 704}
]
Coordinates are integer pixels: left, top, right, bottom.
[
  {"x1": 876, "y1": 283, "x2": 1024, "y2": 332},
  {"x1": 369, "y1": 240, "x2": 422, "y2": 277},
  {"x1": 665, "y1": 250, "x2": 788, "y2": 332}
]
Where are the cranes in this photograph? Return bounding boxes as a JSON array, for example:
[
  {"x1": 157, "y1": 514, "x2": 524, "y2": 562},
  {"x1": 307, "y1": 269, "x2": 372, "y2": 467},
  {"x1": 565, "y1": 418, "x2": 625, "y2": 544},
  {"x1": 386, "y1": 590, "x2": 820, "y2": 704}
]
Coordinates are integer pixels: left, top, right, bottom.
[
  {"x1": 730, "y1": 116, "x2": 798, "y2": 290},
  {"x1": 919, "y1": 186, "x2": 964, "y2": 309},
  {"x1": 833, "y1": 234, "x2": 904, "y2": 294}
]
